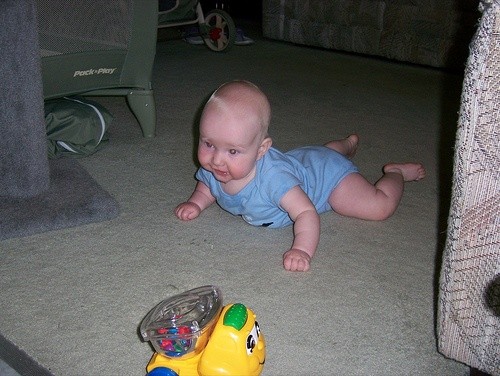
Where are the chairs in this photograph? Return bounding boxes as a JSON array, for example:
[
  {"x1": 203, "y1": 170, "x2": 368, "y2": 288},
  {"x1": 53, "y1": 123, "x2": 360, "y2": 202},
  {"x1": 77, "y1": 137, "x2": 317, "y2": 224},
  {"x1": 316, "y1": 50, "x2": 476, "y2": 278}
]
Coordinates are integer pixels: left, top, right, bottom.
[{"x1": 37, "y1": 0, "x2": 159, "y2": 138}]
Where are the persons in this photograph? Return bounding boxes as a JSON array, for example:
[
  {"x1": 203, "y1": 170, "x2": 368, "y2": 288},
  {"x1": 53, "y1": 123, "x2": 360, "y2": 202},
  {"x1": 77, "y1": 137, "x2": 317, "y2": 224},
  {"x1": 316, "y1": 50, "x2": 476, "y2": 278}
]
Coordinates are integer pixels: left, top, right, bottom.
[{"x1": 174, "y1": 81, "x2": 426, "y2": 272}]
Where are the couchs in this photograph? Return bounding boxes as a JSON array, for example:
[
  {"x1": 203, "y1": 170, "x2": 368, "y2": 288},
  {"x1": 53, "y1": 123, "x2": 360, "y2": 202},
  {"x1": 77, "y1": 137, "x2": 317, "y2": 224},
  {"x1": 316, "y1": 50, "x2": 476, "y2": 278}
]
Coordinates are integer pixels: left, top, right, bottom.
[{"x1": 262, "y1": 0, "x2": 480, "y2": 67}]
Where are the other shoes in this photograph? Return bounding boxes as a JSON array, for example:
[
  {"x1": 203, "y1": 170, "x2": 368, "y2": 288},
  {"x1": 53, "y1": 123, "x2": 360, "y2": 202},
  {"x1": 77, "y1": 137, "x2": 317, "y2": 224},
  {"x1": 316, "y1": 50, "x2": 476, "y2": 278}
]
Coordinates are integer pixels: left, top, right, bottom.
[
  {"x1": 225, "y1": 28, "x2": 256, "y2": 45},
  {"x1": 180, "y1": 28, "x2": 204, "y2": 45}
]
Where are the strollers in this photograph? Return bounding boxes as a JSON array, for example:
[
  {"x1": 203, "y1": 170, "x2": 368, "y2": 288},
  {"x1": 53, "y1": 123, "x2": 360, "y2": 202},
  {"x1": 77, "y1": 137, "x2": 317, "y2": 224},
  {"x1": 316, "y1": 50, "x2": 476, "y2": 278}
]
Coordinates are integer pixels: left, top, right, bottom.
[{"x1": 156, "y1": 0, "x2": 235, "y2": 53}]
